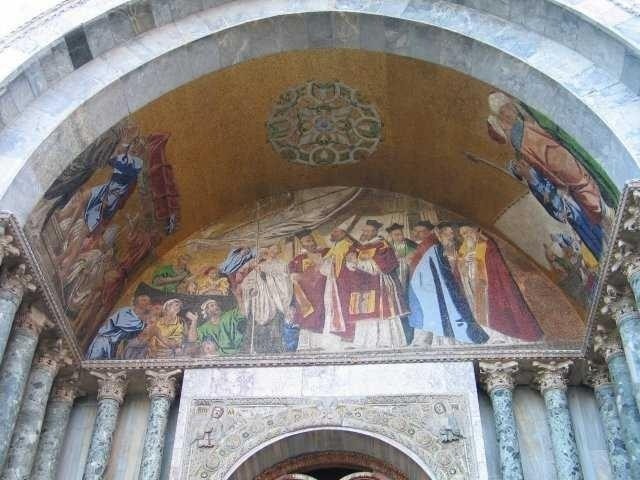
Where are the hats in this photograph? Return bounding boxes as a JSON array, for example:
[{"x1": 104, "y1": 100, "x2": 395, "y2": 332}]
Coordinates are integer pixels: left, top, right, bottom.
[{"x1": 294, "y1": 220, "x2": 474, "y2": 240}]
